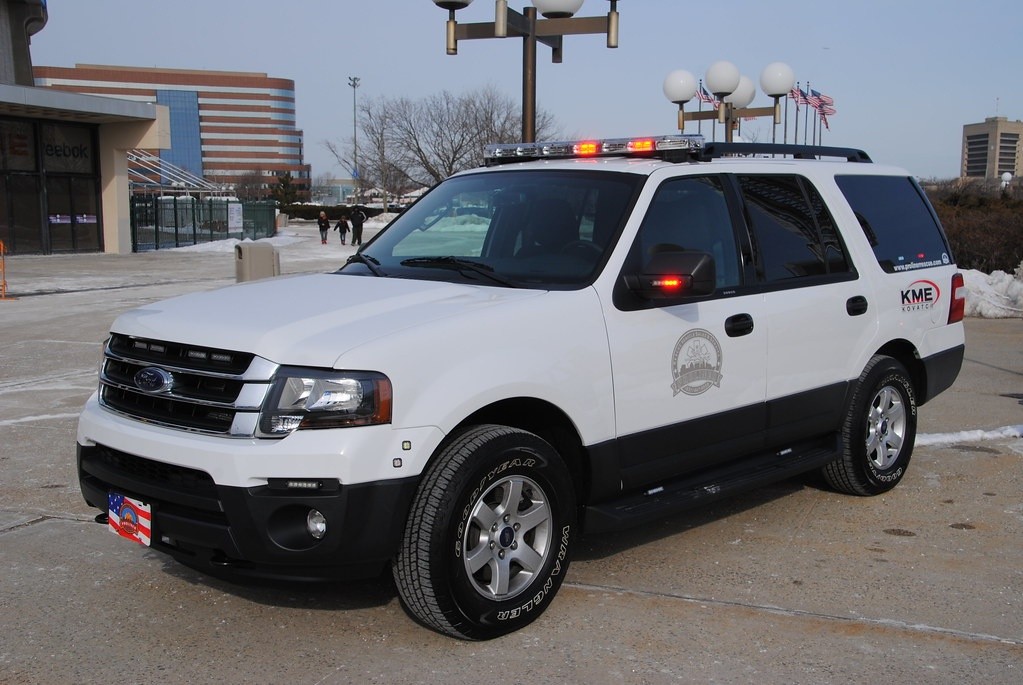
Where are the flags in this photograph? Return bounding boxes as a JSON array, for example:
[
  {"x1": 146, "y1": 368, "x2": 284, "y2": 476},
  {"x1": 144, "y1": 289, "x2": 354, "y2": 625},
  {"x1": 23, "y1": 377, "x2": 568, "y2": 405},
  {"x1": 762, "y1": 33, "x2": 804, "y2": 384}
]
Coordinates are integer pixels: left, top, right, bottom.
[
  {"x1": 695, "y1": 86, "x2": 721, "y2": 110},
  {"x1": 788, "y1": 85, "x2": 836, "y2": 130}
]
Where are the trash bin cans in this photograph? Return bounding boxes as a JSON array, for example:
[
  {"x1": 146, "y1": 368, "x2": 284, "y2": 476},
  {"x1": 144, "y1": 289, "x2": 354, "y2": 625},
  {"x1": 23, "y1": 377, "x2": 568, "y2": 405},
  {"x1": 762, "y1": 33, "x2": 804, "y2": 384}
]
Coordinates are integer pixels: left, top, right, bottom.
[{"x1": 233, "y1": 240, "x2": 281, "y2": 283}]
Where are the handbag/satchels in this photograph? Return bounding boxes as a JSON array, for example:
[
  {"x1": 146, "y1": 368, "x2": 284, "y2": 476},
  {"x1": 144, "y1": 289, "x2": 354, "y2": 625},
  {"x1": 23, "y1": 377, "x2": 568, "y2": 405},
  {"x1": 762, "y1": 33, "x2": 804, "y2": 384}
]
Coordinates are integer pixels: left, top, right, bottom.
[{"x1": 325, "y1": 221, "x2": 330, "y2": 229}]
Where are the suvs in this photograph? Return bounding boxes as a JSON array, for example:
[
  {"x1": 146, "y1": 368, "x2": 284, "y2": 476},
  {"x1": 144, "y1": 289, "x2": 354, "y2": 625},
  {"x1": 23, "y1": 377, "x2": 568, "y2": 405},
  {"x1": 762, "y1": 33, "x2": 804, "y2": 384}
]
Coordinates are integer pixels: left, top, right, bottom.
[{"x1": 73, "y1": 133, "x2": 972, "y2": 641}]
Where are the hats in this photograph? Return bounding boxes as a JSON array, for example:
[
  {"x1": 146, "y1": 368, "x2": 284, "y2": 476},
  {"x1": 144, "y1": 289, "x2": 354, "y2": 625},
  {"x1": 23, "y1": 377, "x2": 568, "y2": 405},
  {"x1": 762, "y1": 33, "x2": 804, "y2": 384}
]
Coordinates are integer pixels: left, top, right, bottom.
[{"x1": 341, "y1": 216, "x2": 346, "y2": 219}]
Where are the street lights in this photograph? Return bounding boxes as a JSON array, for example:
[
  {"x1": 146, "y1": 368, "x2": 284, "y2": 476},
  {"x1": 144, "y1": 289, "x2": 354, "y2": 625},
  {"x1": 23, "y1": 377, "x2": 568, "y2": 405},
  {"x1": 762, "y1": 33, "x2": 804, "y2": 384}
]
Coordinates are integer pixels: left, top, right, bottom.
[
  {"x1": 433, "y1": 0, "x2": 621, "y2": 143},
  {"x1": 663, "y1": 58, "x2": 797, "y2": 157},
  {"x1": 347, "y1": 75, "x2": 362, "y2": 206}
]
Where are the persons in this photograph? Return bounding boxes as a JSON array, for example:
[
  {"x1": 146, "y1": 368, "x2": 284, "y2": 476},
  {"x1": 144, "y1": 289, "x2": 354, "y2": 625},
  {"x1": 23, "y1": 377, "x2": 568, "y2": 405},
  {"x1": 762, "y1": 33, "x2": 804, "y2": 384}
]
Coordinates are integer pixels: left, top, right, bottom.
[
  {"x1": 334, "y1": 215, "x2": 351, "y2": 246},
  {"x1": 318, "y1": 211, "x2": 331, "y2": 245},
  {"x1": 350, "y1": 203, "x2": 367, "y2": 247}
]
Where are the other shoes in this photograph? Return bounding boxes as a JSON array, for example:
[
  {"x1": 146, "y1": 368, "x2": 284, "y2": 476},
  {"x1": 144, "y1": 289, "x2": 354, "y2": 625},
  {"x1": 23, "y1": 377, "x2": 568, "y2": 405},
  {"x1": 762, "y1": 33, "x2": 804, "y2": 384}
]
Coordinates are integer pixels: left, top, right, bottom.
[
  {"x1": 352, "y1": 243, "x2": 355, "y2": 246},
  {"x1": 325, "y1": 240, "x2": 327, "y2": 244},
  {"x1": 322, "y1": 240, "x2": 324, "y2": 244},
  {"x1": 343, "y1": 242, "x2": 345, "y2": 245},
  {"x1": 358, "y1": 242, "x2": 361, "y2": 245}
]
